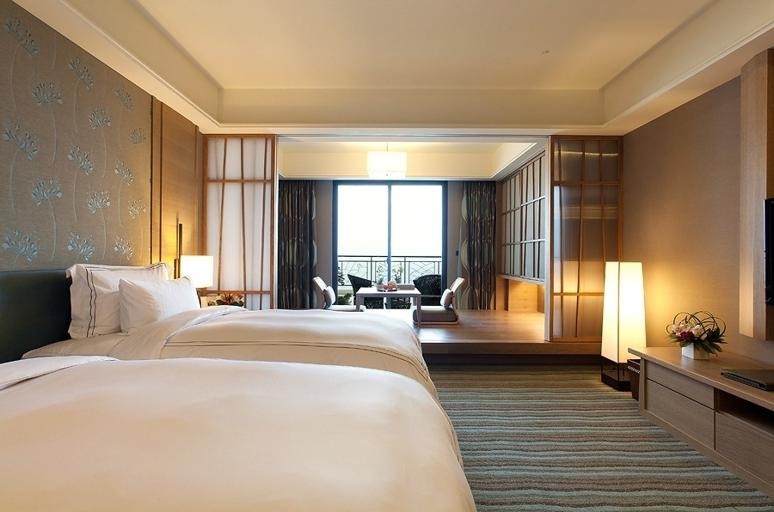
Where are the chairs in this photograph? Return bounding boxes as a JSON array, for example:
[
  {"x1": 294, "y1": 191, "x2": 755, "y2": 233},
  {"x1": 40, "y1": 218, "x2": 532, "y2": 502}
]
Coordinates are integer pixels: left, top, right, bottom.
[
  {"x1": 413, "y1": 275, "x2": 442, "y2": 306},
  {"x1": 347, "y1": 273, "x2": 384, "y2": 308},
  {"x1": 312, "y1": 276, "x2": 366, "y2": 312},
  {"x1": 413, "y1": 277, "x2": 466, "y2": 326}
]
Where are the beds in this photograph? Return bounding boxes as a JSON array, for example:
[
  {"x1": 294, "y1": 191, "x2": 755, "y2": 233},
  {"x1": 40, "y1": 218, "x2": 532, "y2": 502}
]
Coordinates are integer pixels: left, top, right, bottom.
[
  {"x1": 23, "y1": 270, "x2": 439, "y2": 402},
  {"x1": 0, "y1": 354, "x2": 477, "y2": 509}
]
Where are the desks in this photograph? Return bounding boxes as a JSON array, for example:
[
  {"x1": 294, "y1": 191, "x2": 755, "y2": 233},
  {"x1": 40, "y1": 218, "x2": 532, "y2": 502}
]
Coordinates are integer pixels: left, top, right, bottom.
[{"x1": 355, "y1": 285, "x2": 422, "y2": 329}]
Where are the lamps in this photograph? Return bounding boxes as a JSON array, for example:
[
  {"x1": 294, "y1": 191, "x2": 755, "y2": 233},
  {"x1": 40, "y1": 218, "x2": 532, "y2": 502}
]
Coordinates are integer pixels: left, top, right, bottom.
[
  {"x1": 599, "y1": 261, "x2": 647, "y2": 391},
  {"x1": 180, "y1": 254, "x2": 213, "y2": 288}
]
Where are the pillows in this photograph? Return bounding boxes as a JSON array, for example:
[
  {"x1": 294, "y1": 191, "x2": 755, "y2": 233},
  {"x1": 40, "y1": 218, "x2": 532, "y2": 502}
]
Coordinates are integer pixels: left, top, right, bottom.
[{"x1": 67, "y1": 262, "x2": 200, "y2": 340}]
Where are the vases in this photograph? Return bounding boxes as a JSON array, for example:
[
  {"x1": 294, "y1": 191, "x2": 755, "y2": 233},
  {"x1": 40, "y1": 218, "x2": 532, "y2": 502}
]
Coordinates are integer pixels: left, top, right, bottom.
[
  {"x1": 664, "y1": 312, "x2": 726, "y2": 356},
  {"x1": 680, "y1": 344, "x2": 711, "y2": 360}
]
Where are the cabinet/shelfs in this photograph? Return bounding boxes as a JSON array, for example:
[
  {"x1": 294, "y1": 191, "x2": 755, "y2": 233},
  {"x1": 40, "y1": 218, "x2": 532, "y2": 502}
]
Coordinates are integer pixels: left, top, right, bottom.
[
  {"x1": 496, "y1": 150, "x2": 545, "y2": 285},
  {"x1": 628, "y1": 347, "x2": 774, "y2": 496}
]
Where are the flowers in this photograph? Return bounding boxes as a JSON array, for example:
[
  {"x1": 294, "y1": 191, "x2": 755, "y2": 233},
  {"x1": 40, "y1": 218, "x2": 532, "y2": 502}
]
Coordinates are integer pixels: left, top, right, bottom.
[{"x1": 215, "y1": 290, "x2": 243, "y2": 307}]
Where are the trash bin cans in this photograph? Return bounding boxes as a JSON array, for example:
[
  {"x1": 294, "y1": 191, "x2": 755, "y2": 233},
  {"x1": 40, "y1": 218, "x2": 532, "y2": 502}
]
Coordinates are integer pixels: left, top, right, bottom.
[{"x1": 627, "y1": 359, "x2": 640, "y2": 400}]
